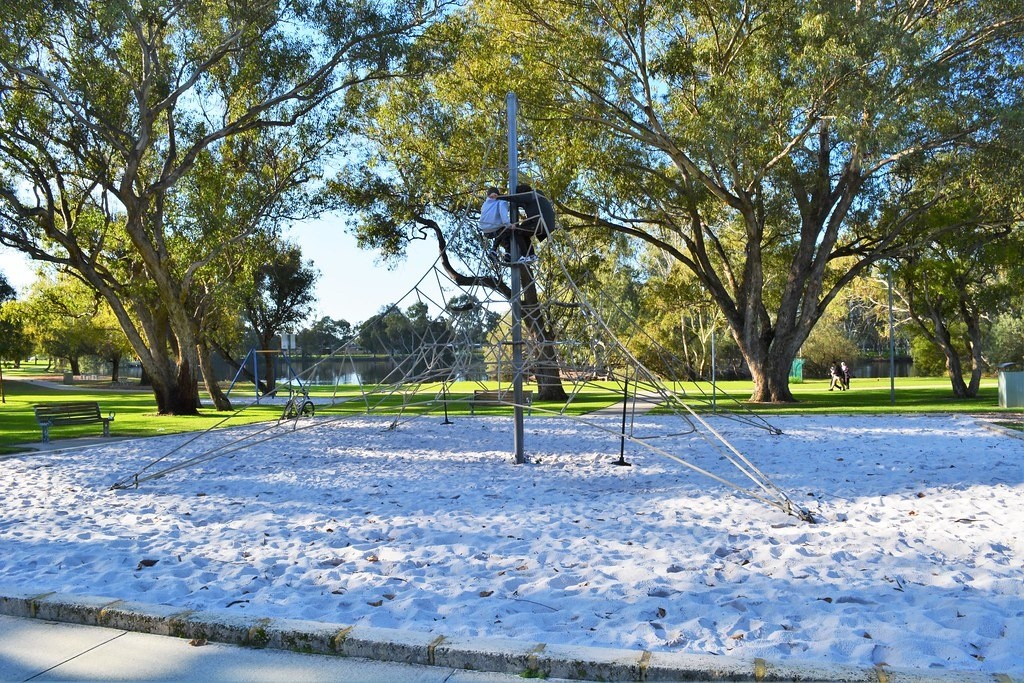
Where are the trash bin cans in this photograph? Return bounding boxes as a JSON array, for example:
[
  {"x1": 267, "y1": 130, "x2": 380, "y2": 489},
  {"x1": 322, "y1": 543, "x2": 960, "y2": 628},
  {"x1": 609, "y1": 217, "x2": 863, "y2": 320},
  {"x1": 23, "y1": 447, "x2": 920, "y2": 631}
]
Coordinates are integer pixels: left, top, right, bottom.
[
  {"x1": 63, "y1": 369, "x2": 74, "y2": 385},
  {"x1": 995, "y1": 361, "x2": 1024, "y2": 408}
]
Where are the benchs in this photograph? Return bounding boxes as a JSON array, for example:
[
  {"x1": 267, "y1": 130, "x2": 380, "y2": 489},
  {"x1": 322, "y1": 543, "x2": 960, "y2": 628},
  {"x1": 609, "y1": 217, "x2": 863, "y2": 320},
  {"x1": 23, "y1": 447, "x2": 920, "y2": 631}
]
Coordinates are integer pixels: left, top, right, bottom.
[
  {"x1": 33, "y1": 401, "x2": 115, "y2": 444},
  {"x1": 466, "y1": 390, "x2": 533, "y2": 416}
]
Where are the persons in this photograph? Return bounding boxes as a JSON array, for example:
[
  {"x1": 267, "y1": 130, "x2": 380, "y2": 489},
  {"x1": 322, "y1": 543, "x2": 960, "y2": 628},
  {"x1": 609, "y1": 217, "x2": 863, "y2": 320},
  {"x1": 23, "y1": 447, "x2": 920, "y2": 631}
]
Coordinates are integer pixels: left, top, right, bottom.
[
  {"x1": 830, "y1": 367, "x2": 842, "y2": 391},
  {"x1": 841, "y1": 362, "x2": 850, "y2": 390},
  {"x1": 828, "y1": 362, "x2": 847, "y2": 391},
  {"x1": 487, "y1": 184, "x2": 556, "y2": 262},
  {"x1": 478, "y1": 187, "x2": 513, "y2": 264}
]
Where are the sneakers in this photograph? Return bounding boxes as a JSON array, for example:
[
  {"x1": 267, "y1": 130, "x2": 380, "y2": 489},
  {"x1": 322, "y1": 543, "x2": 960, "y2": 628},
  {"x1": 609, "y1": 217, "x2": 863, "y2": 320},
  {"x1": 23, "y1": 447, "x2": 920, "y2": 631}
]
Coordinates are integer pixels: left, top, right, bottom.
[
  {"x1": 517, "y1": 255, "x2": 539, "y2": 263},
  {"x1": 487, "y1": 251, "x2": 502, "y2": 263},
  {"x1": 503, "y1": 253, "x2": 511, "y2": 263}
]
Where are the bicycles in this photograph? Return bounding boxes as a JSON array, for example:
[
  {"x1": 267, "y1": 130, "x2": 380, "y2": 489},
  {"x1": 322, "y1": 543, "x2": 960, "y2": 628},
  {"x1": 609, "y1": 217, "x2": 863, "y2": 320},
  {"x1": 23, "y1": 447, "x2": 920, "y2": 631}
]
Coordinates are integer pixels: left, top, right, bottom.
[{"x1": 280, "y1": 386, "x2": 315, "y2": 420}]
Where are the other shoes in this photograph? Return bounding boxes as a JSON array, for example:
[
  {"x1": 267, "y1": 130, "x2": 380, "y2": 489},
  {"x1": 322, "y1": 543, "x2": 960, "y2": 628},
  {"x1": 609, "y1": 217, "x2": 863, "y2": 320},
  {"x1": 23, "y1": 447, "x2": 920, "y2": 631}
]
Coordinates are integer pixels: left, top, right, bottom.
[{"x1": 829, "y1": 387, "x2": 850, "y2": 391}]
